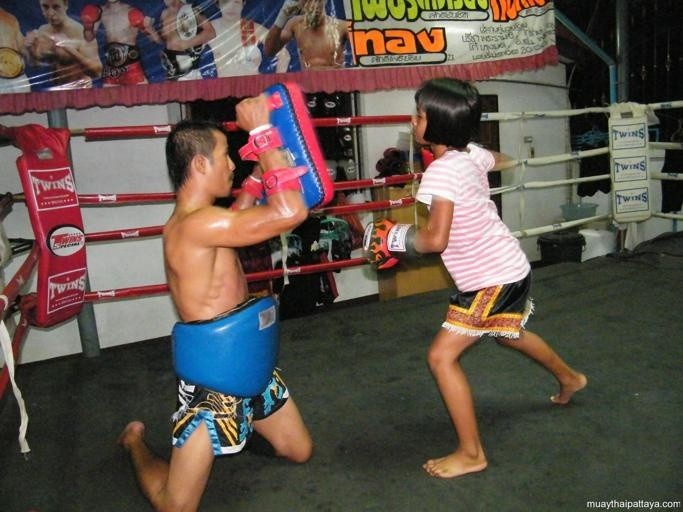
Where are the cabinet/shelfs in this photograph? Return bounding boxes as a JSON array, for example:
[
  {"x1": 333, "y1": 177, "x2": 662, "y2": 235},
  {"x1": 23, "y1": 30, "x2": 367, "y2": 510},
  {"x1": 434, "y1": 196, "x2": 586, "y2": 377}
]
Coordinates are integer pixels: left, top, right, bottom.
[{"x1": 371, "y1": 185, "x2": 453, "y2": 301}]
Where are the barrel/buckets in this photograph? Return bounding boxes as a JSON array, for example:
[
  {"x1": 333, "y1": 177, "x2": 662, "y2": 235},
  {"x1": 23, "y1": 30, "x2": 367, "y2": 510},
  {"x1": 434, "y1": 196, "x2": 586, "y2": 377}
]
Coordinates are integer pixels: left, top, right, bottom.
[{"x1": 537, "y1": 231, "x2": 587, "y2": 265}]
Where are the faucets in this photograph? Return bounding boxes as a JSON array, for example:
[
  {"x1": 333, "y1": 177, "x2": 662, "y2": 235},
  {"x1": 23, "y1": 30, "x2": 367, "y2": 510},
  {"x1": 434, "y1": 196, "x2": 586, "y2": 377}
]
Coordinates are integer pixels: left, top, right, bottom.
[{"x1": 573, "y1": 193, "x2": 581, "y2": 206}]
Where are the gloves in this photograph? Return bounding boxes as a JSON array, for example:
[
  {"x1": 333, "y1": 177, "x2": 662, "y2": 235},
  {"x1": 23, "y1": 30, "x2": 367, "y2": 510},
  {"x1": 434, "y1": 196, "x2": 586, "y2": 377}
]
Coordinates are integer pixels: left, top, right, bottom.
[
  {"x1": 127, "y1": 8, "x2": 147, "y2": 33},
  {"x1": 362, "y1": 217, "x2": 423, "y2": 270},
  {"x1": 79, "y1": 3, "x2": 103, "y2": 29},
  {"x1": 420, "y1": 145, "x2": 435, "y2": 171},
  {"x1": 273, "y1": 1, "x2": 304, "y2": 30}
]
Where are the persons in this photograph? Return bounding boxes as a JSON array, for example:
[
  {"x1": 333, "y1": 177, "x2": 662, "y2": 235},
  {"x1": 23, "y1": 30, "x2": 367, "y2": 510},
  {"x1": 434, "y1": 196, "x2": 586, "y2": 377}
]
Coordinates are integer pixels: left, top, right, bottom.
[
  {"x1": 262, "y1": 0, "x2": 352, "y2": 72},
  {"x1": 25, "y1": 0, "x2": 105, "y2": 87},
  {"x1": 361, "y1": 71, "x2": 588, "y2": 480},
  {"x1": 207, "y1": 0, "x2": 291, "y2": 77},
  {"x1": 0, "y1": 7, "x2": 31, "y2": 93},
  {"x1": 143, "y1": 0, "x2": 216, "y2": 81},
  {"x1": 120, "y1": 92, "x2": 316, "y2": 512},
  {"x1": 82, "y1": 0, "x2": 150, "y2": 86}
]
